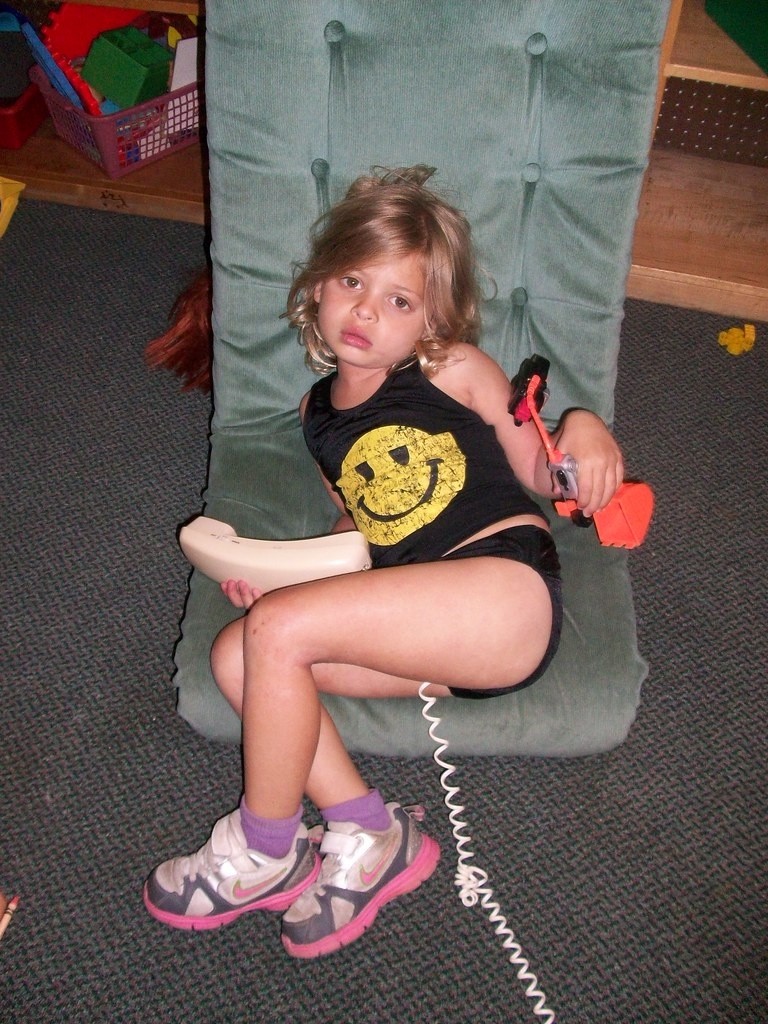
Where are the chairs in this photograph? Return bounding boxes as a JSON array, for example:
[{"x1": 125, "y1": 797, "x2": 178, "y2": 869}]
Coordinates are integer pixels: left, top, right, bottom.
[{"x1": 170, "y1": 0, "x2": 672, "y2": 762}]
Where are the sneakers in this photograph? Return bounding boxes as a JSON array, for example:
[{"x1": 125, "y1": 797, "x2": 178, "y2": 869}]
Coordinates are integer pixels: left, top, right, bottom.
[
  {"x1": 144, "y1": 811, "x2": 321, "y2": 931},
  {"x1": 282, "y1": 803, "x2": 441, "y2": 958}
]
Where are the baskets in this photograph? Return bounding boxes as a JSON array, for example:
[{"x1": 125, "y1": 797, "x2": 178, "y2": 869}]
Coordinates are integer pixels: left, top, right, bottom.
[{"x1": 28, "y1": 64, "x2": 203, "y2": 180}]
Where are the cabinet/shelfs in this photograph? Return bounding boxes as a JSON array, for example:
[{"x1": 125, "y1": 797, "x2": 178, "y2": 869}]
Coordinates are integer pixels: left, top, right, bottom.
[{"x1": 0, "y1": 0, "x2": 768, "y2": 323}]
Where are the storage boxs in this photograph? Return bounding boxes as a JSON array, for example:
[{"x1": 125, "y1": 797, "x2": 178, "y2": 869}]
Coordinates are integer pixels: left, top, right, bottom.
[
  {"x1": 29, "y1": 10, "x2": 207, "y2": 179},
  {"x1": 0, "y1": 30, "x2": 48, "y2": 149},
  {"x1": 80, "y1": 26, "x2": 175, "y2": 111}
]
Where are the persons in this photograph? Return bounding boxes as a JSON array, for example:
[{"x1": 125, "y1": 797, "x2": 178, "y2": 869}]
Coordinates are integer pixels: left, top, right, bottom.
[{"x1": 142, "y1": 160, "x2": 624, "y2": 958}]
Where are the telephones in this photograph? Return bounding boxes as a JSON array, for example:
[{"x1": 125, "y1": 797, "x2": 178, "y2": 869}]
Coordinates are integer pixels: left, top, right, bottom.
[{"x1": 176, "y1": 517, "x2": 375, "y2": 593}]
